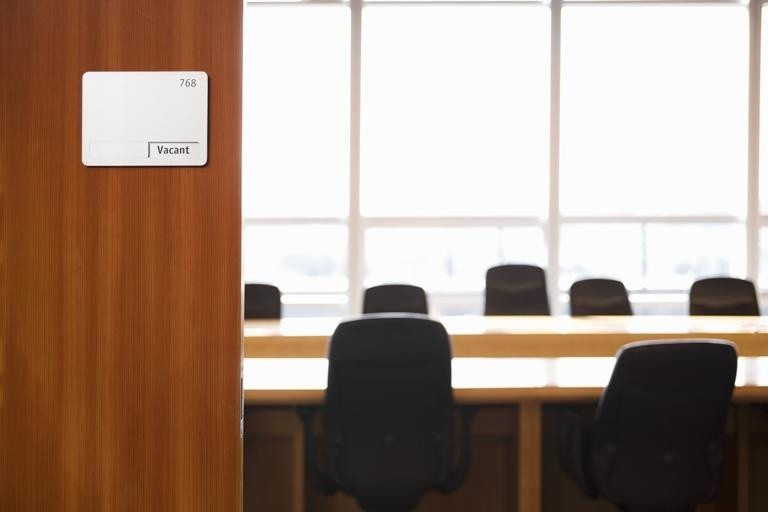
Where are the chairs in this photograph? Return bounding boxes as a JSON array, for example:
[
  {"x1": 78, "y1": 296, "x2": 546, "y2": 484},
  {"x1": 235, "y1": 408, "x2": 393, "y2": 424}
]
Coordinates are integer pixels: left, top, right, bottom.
[{"x1": 244, "y1": 264, "x2": 760, "y2": 511}]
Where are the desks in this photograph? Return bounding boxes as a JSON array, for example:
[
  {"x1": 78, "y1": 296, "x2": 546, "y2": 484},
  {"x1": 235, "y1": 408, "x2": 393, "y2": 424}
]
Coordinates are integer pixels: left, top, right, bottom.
[{"x1": 242, "y1": 312, "x2": 767, "y2": 511}]
